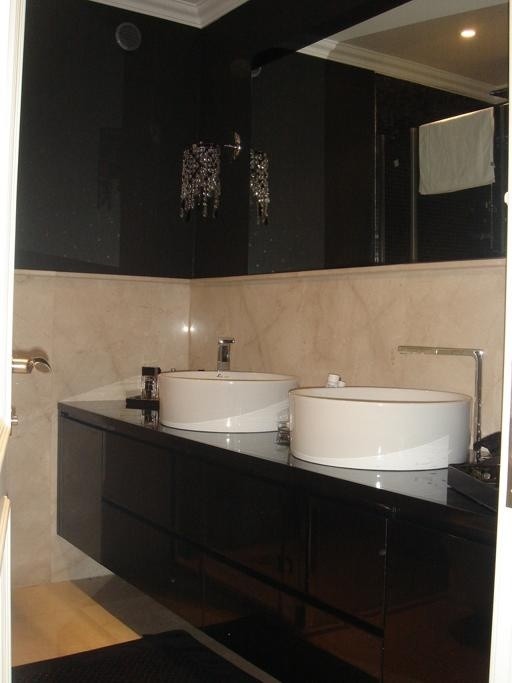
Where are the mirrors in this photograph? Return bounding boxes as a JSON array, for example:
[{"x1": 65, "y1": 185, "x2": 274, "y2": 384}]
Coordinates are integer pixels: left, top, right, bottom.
[{"x1": 247, "y1": 0, "x2": 508, "y2": 276}]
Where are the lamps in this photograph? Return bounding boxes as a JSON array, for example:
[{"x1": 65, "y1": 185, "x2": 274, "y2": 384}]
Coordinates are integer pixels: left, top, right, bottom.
[
  {"x1": 248, "y1": 148, "x2": 271, "y2": 228},
  {"x1": 179, "y1": 130, "x2": 242, "y2": 223}
]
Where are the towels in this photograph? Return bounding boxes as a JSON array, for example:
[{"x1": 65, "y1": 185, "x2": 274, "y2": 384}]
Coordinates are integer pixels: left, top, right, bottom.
[{"x1": 419, "y1": 104, "x2": 496, "y2": 196}]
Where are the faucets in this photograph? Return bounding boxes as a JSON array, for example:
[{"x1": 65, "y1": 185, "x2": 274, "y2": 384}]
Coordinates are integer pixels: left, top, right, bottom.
[
  {"x1": 398, "y1": 345, "x2": 484, "y2": 462},
  {"x1": 217, "y1": 336, "x2": 235, "y2": 371}
]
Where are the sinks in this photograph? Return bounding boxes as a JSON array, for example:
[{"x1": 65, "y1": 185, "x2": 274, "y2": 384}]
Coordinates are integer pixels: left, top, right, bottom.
[
  {"x1": 156, "y1": 370, "x2": 300, "y2": 433},
  {"x1": 288, "y1": 386, "x2": 473, "y2": 472}
]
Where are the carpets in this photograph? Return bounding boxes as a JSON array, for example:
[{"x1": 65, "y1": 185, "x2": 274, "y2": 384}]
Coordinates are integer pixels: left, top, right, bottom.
[{"x1": 12, "y1": 629, "x2": 263, "y2": 683}]
